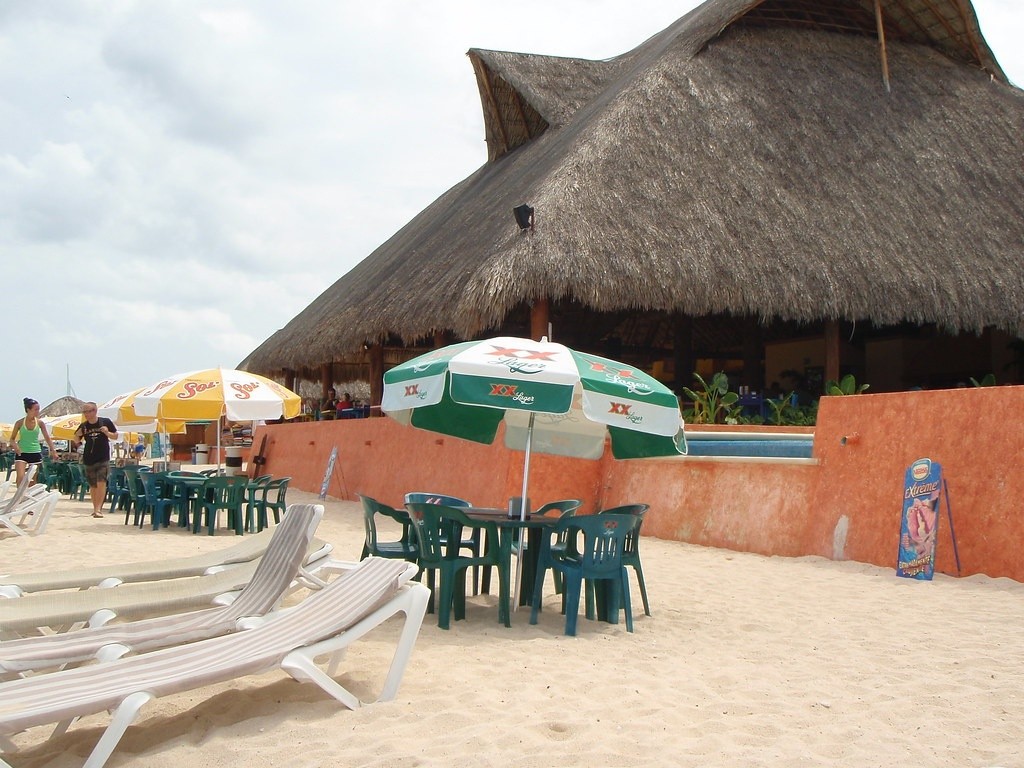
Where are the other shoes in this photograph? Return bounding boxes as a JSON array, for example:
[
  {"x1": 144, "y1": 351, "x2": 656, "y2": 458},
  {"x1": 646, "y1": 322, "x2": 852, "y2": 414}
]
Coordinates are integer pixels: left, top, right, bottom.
[
  {"x1": 90, "y1": 511, "x2": 103, "y2": 517},
  {"x1": 27, "y1": 511, "x2": 34, "y2": 515}
]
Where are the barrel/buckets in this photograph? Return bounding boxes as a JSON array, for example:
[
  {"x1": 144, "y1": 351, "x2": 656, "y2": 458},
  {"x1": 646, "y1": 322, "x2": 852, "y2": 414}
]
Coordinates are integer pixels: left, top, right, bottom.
[
  {"x1": 191, "y1": 444, "x2": 208, "y2": 465},
  {"x1": 225, "y1": 445, "x2": 242, "y2": 477}
]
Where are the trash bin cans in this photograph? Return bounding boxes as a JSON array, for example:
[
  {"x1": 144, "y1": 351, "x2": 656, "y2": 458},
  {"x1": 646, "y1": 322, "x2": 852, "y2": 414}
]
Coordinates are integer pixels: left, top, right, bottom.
[
  {"x1": 196, "y1": 444, "x2": 208, "y2": 465},
  {"x1": 224, "y1": 446, "x2": 243, "y2": 476},
  {"x1": 191, "y1": 447, "x2": 196, "y2": 465}
]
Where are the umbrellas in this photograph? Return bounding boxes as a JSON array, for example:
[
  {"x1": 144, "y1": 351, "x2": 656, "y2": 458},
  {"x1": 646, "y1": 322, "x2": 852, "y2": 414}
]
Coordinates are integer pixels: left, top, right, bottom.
[
  {"x1": 0, "y1": 391, "x2": 189, "y2": 471},
  {"x1": 380, "y1": 336, "x2": 688, "y2": 611},
  {"x1": 134, "y1": 366, "x2": 301, "y2": 530}
]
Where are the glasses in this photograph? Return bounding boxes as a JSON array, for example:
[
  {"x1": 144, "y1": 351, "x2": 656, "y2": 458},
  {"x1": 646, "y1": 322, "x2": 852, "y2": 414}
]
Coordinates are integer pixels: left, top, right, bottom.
[{"x1": 82, "y1": 409, "x2": 94, "y2": 413}]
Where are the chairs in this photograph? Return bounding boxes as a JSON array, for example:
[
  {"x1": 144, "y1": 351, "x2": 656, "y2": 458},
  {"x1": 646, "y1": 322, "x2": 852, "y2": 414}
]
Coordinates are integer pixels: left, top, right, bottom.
[{"x1": 0, "y1": 451, "x2": 651, "y2": 768}]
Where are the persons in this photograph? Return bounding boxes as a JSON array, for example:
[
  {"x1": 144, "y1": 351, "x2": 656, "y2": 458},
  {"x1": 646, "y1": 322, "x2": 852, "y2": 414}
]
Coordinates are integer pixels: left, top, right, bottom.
[
  {"x1": 10, "y1": 397, "x2": 59, "y2": 515},
  {"x1": 322, "y1": 388, "x2": 339, "y2": 414},
  {"x1": 134, "y1": 433, "x2": 144, "y2": 458},
  {"x1": 74, "y1": 402, "x2": 119, "y2": 518},
  {"x1": 336, "y1": 393, "x2": 352, "y2": 419}
]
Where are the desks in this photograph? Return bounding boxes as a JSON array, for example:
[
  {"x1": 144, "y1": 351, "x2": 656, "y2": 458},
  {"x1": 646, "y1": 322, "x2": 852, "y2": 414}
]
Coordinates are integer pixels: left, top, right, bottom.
[
  {"x1": 441, "y1": 506, "x2": 560, "y2": 613},
  {"x1": 186, "y1": 481, "x2": 258, "y2": 534}
]
